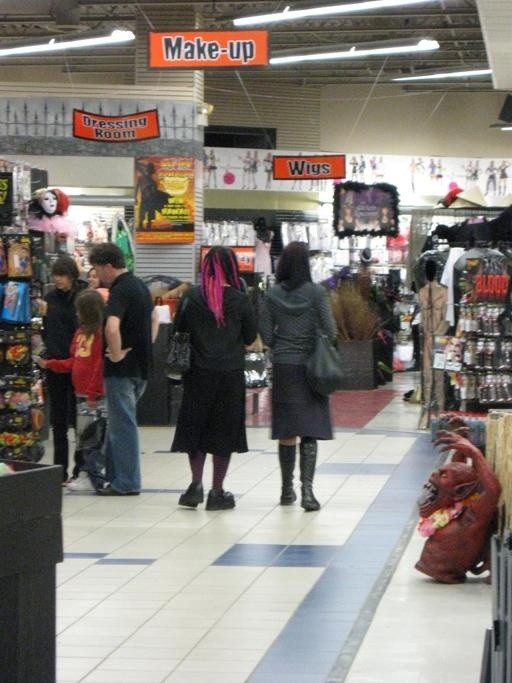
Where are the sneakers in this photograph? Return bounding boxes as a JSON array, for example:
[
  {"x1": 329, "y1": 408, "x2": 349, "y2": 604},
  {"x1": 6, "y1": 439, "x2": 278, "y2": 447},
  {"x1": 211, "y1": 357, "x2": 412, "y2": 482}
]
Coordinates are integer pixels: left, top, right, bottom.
[{"x1": 67, "y1": 471, "x2": 95, "y2": 490}]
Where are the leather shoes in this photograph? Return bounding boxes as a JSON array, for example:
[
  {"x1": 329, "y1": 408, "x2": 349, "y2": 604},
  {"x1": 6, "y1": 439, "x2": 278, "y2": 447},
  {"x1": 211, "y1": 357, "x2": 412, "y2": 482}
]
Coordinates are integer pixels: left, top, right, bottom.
[
  {"x1": 205, "y1": 491, "x2": 236, "y2": 510},
  {"x1": 98, "y1": 483, "x2": 139, "y2": 495},
  {"x1": 178, "y1": 483, "x2": 203, "y2": 508}
]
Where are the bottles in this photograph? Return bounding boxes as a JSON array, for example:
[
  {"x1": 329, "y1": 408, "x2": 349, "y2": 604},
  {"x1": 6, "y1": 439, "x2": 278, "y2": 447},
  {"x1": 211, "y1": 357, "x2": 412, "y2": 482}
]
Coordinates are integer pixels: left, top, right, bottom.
[{"x1": 439, "y1": 301, "x2": 512, "y2": 405}]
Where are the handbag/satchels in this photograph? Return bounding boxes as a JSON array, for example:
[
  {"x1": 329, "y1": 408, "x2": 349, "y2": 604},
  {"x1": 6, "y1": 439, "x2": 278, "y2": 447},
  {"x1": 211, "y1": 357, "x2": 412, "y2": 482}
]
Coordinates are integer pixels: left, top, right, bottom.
[
  {"x1": 167, "y1": 331, "x2": 191, "y2": 374},
  {"x1": 305, "y1": 330, "x2": 345, "y2": 397}
]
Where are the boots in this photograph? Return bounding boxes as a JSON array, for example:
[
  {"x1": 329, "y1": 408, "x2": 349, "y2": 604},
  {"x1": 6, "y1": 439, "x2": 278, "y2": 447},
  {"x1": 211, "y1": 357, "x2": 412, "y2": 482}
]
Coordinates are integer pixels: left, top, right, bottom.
[
  {"x1": 299, "y1": 441, "x2": 321, "y2": 510},
  {"x1": 278, "y1": 443, "x2": 296, "y2": 505}
]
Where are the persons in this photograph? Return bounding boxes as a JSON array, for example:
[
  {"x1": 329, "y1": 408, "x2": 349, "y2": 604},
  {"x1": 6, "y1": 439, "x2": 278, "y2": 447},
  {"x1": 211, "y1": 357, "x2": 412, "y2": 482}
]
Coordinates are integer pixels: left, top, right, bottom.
[
  {"x1": 38, "y1": 190, "x2": 59, "y2": 214},
  {"x1": 30, "y1": 287, "x2": 112, "y2": 495},
  {"x1": 414, "y1": 428, "x2": 503, "y2": 582},
  {"x1": 134, "y1": 162, "x2": 175, "y2": 231},
  {"x1": 258, "y1": 239, "x2": 348, "y2": 512},
  {"x1": 35, "y1": 257, "x2": 90, "y2": 486},
  {"x1": 412, "y1": 258, "x2": 451, "y2": 431},
  {"x1": 167, "y1": 244, "x2": 257, "y2": 512},
  {"x1": 86, "y1": 266, "x2": 110, "y2": 305},
  {"x1": 88, "y1": 240, "x2": 160, "y2": 495}
]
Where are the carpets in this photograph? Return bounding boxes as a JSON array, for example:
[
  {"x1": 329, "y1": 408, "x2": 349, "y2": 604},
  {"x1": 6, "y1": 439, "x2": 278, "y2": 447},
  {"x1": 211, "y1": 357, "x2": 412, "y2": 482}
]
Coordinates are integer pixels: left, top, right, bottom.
[{"x1": 223, "y1": 383, "x2": 403, "y2": 430}]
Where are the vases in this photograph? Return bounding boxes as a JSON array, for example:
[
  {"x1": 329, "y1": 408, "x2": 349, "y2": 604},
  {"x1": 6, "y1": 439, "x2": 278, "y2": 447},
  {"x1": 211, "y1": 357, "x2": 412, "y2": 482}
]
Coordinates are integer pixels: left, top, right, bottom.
[{"x1": 329, "y1": 338, "x2": 378, "y2": 388}]
